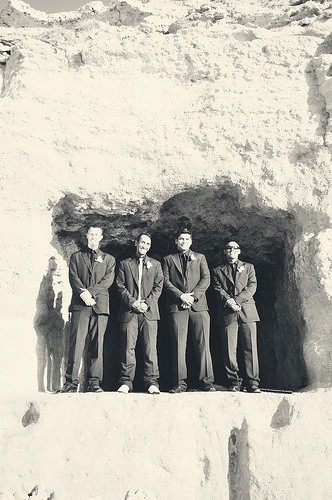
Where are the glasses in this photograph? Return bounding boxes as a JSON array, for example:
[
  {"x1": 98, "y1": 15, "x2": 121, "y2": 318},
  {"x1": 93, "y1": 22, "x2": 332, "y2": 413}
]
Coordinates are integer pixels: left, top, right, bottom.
[{"x1": 224, "y1": 246, "x2": 240, "y2": 249}]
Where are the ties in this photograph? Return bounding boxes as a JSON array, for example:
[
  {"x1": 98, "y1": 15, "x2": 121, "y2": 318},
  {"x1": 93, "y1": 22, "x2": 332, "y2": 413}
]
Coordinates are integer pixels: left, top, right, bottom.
[
  {"x1": 181, "y1": 253, "x2": 187, "y2": 276},
  {"x1": 230, "y1": 263, "x2": 236, "y2": 284},
  {"x1": 138, "y1": 258, "x2": 143, "y2": 300},
  {"x1": 91, "y1": 251, "x2": 95, "y2": 269}
]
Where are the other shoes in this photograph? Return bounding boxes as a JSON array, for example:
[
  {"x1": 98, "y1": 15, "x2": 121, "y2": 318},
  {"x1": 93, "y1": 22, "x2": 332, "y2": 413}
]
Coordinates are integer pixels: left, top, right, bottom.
[
  {"x1": 148, "y1": 385, "x2": 160, "y2": 394},
  {"x1": 117, "y1": 385, "x2": 130, "y2": 393}
]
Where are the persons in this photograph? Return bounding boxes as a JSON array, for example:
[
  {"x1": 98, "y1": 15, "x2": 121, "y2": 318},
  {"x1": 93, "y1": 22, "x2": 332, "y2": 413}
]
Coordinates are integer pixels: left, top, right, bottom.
[
  {"x1": 211, "y1": 241, "x2": 262, "y2": 393},
  {"x1": 116, "y1": 232, "x2": 164, "y2": 394},
  {"x1": 57, "y1": 223, "x2": 116, "y2": 393},
  {"x1": 161, "y1": 229, "x2": 216, "y2": 393}
]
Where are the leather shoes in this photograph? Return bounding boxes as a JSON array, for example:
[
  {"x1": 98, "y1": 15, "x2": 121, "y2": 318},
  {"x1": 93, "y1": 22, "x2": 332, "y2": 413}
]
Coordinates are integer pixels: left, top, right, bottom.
[
  {"x1": 169, "y1": 384, "x2": 187, "y2": 392},
  {"x1": 85, "y1": 385, "x2": 104, "y2": 392},
  {"x1": 228, "y1": 384, "x2": 241, "y2": 391},
  {"x1": 243, "y1": 385, "x2": 260, "y2": 392},
  {"x1": 56, "y1": 384, "x2": 78, "y2": 393},
  {"x1": 198, "y1": 382, "x2": 216, "y2": 391}
]
